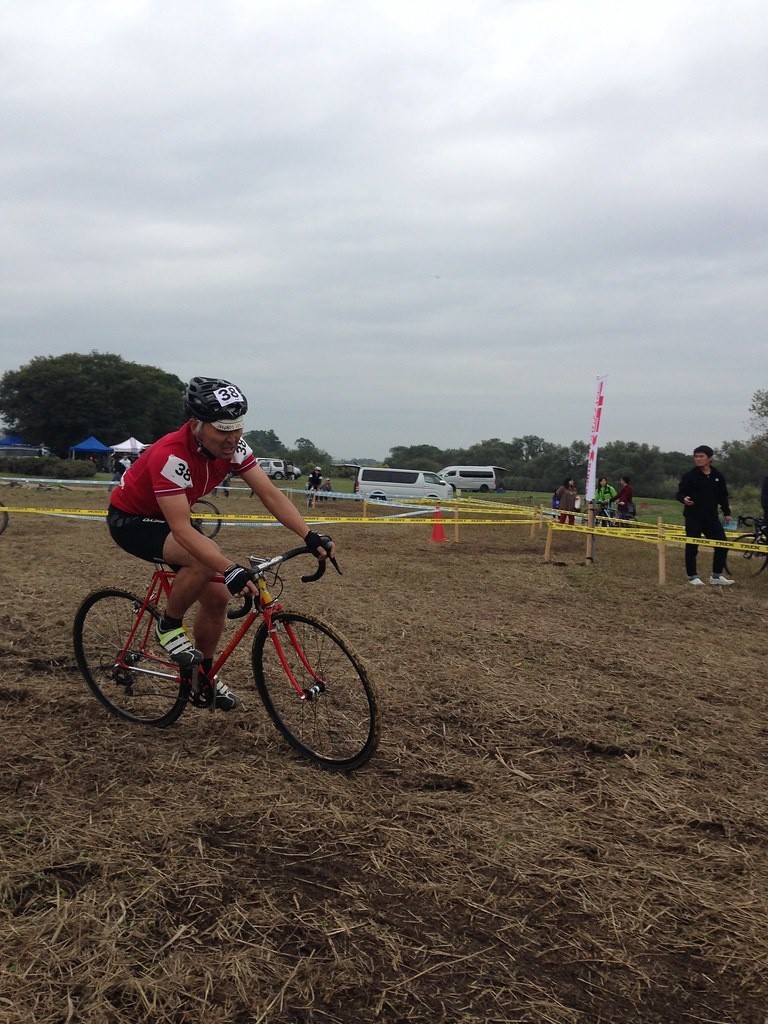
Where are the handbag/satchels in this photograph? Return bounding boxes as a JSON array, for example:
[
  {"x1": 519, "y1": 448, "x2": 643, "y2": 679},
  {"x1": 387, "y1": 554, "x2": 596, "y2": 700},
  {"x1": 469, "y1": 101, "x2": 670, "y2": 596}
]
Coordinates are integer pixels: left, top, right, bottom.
[{"x1": 627, "y1": 503, "x2": 637, "y2": 513}]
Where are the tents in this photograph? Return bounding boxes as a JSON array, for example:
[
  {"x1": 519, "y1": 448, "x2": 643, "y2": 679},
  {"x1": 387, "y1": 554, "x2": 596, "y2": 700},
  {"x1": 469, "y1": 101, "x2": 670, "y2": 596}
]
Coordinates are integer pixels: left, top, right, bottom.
[{"x1": 70, "y1": 437, "x2": 145, "y2": 471}]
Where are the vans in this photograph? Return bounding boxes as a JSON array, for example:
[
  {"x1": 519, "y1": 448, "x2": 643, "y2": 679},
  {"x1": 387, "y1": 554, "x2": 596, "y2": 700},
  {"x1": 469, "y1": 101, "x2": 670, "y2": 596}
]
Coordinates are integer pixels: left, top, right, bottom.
[
  {"x1": 254, "y1": 458, "x2": 293, "y2": 480},
  {"x1": 436, "y1": 465, "x2": 510, "y2": 493},
  {"x1": 332, "y1": 463, "x2": 453, "y2": 502}
]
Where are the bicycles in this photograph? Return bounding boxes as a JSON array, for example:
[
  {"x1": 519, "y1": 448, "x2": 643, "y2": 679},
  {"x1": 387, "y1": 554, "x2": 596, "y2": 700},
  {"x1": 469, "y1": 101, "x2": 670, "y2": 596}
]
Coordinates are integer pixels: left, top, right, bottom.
[
  {"x1": 582, "y1": 501, "x2": 640, "y2": 528},
  {"x1": 724, "y1": 515, "x2": 768, "y2": 577},
  {"x1": 190, "y1": 500, "x2": 221, "y2": 538},
  {"x1": 73, "y1": 546, "x2": 382, "y2": 772}
]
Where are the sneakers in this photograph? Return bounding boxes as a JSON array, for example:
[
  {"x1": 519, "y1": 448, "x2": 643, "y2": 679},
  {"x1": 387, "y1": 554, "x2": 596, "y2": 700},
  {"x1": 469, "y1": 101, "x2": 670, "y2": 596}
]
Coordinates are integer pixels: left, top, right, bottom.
[
  {"x1": 689, "y1": 577, "x2": 706, "y2": 585},
  {"x1": 155, "y1": 619, "x2": 204, "y2": 663},
  {"x1": 201, "y1": 674, "x2": 240, "y2": 709},
  {"x1": 709, "y1": 576, "x2": 735, "y2": 585}
]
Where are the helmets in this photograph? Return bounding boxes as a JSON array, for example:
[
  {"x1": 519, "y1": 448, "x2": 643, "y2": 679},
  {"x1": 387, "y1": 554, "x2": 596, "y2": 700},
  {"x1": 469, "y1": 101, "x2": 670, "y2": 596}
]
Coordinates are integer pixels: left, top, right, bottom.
[
  {"x1": 315, "y1": 467, "x2": 322, "y2": 471},
  {"x1": 185, "y1": 377, "x2": 248, "y2": 421}
]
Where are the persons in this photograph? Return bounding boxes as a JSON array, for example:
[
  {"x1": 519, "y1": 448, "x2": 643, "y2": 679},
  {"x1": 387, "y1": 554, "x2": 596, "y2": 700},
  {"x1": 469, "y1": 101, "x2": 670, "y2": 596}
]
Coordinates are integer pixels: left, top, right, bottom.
[
  {"x1": 210, "y1": 469, "x2": 231, "y2": 497},
  {"x1": 556, "y1": 478, "x2": 580, "y2": 526},
  {"x1": 594, "y1": 476, "x2": 617, "y2": 526},
  {"x1": 676, "y1": 445, "x2": 736, "y2": 585},
  {"x1": 602, "y1": 475, "x2": 633, "y2": 527},
  {"x1": 107, "y1": 378, "x2": 337, "y2": 711},
  {"x1": 305, "y1": 468, "x2": 333, "y2": 506},
  {"x1": 86, "y1": 453, "x2": 141, "y2": 490}
]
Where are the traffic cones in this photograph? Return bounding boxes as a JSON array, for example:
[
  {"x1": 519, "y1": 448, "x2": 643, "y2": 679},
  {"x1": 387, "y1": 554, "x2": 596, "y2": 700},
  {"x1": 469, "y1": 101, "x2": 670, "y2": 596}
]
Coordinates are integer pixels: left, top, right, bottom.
[{"x1": 426, "y1": 505, "x2": 450, "y2": 543}]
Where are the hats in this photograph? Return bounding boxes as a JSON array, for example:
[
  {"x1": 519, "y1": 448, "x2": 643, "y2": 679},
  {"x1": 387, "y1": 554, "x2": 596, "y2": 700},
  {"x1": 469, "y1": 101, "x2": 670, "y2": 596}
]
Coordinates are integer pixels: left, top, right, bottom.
[{"x1": 194, "y1": 394, "x2": 244, "y2": 431}]
